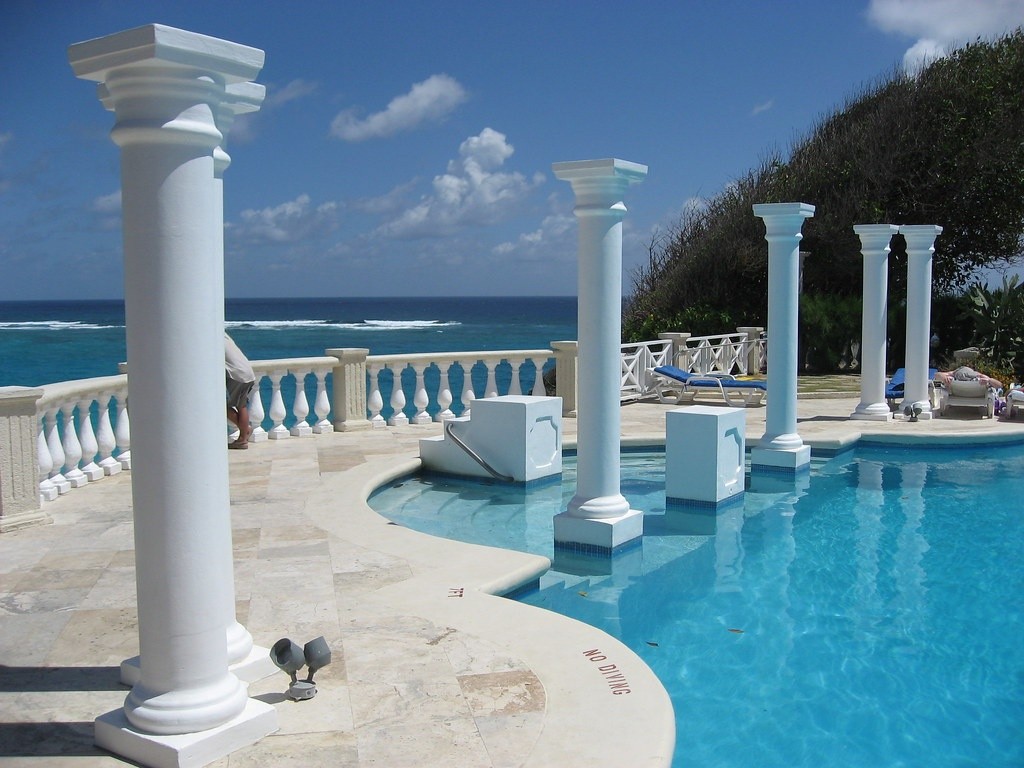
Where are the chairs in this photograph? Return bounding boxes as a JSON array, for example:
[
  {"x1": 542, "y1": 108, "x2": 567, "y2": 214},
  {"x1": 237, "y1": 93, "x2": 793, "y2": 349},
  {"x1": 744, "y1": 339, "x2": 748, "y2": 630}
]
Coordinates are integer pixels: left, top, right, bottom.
[
  {"x1": 885, "y1": 368, "x2": 939, "y2": 408},
  {"x1": 1006, "y1": 382, "x2": 1024, "y2": 419},
  {"x1": 645, "y1": 364, "x2": 767, "y2": 408},
  {"x1": 940, "y1": 381, "x2": 996, "y2": 420}
]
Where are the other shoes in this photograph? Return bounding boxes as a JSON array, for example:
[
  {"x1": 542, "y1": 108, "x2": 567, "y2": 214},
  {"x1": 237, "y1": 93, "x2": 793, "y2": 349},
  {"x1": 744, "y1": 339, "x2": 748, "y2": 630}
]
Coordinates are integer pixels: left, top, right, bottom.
[{"x1": 228, "y1": 425, "x2": 253, "y2": 449}]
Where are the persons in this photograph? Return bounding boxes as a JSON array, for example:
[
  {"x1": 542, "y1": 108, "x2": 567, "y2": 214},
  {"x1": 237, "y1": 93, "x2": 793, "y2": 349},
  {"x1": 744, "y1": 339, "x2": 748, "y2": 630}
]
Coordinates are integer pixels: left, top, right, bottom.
[
  {"x1": 934, "y1": 366, "x2": 1003, "y2": 390},
  {"x1": 225, "y1": 331, "x2": 255, "y2": 449}
]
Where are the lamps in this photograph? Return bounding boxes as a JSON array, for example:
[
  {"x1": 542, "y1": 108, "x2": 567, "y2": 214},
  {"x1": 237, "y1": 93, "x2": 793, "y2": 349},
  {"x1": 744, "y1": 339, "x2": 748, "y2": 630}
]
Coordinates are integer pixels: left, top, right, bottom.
[
  {"x1": 270, "y1": 636, "x2": 334, "y2": 699},
  {"x1": 903, "y1": 402, "x2": 923, "y2": 422}
]
